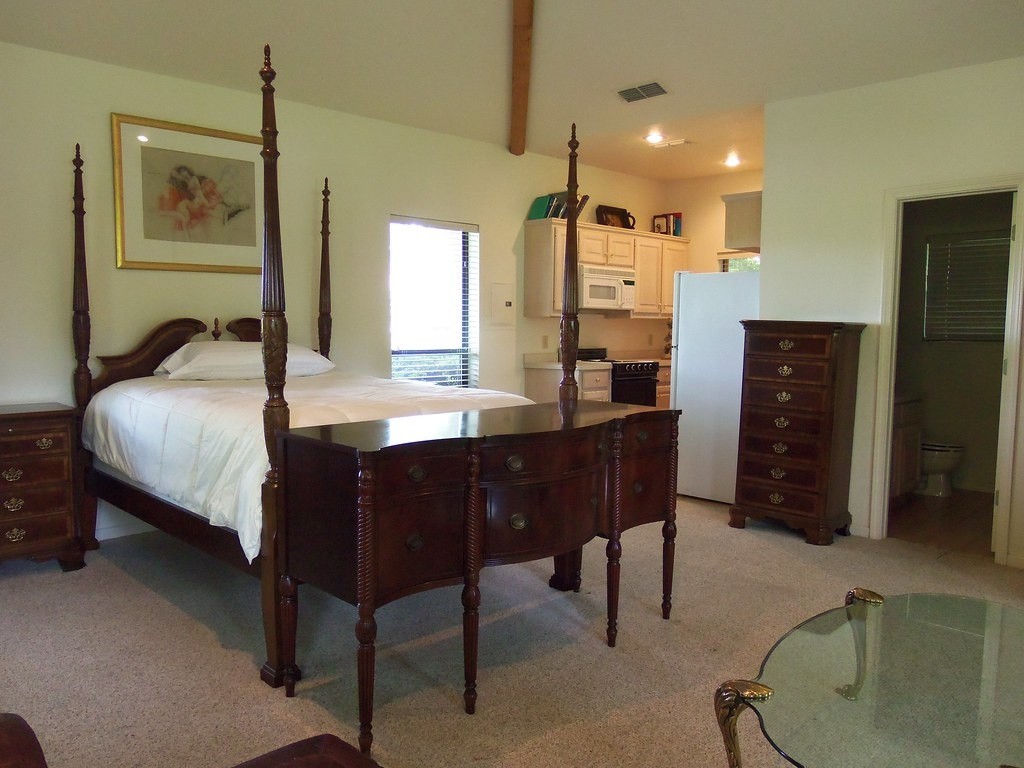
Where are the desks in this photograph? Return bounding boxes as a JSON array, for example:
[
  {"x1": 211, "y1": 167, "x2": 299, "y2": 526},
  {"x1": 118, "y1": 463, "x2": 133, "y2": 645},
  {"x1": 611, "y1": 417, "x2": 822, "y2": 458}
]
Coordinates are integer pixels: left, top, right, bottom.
[{"x1": 712, "y1": 586, "x2": 1024, "y2": 768}]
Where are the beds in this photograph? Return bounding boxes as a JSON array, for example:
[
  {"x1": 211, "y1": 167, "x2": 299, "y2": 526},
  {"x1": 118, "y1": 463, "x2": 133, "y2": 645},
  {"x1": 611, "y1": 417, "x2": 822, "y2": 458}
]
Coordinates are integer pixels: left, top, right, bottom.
[{"x1": 69, "y1": 39, "x2": 592, "y2": 689}]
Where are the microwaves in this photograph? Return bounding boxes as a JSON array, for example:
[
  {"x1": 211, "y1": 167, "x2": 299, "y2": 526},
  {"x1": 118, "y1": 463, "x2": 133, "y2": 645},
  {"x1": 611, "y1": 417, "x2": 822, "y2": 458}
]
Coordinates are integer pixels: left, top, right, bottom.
[{"x1": 577, "y1": 262, "x2": 636, "y2": 310}]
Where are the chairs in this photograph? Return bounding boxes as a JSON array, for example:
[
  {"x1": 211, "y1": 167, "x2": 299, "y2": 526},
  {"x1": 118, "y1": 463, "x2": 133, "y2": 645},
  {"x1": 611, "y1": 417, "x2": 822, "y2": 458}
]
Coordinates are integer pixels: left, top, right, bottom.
[{"x1": 0, "y1": 713, "x2": 381, "y2": 768}]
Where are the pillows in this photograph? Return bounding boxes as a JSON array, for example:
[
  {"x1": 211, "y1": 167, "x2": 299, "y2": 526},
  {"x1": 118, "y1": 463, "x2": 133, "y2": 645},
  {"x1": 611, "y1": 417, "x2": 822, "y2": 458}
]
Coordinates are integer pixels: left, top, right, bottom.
[{"x1": 154, "y1": 341, "x2": 337, "y2": 380}]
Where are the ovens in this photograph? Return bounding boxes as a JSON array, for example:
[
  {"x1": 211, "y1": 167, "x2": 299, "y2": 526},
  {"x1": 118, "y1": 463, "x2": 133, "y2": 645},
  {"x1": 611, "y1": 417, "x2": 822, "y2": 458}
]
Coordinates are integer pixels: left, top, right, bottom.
[{"x1": 611, "y1": 380, "x2": 659, "y2": 407}]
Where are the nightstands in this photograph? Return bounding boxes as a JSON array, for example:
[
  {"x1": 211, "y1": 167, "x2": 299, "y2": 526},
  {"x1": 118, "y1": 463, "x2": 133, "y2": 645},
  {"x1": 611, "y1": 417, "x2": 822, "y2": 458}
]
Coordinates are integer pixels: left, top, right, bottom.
[{"x1": 0, "y1": 402, "x2": 87, "y2": 576}]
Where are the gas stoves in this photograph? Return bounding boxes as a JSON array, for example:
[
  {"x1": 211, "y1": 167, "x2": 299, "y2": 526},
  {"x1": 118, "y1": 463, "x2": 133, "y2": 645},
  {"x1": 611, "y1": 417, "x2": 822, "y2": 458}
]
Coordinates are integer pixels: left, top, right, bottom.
[{"x1": 557, "y1": 347, "x2": 659, "y2": 378}]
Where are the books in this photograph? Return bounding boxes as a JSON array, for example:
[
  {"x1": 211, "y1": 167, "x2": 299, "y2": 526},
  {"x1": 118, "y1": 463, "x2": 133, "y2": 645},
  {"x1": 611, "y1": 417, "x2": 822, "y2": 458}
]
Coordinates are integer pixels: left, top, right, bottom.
[
  {"x1": 590, "y1": 359, "x2": 615, "y2": 362},
  {"x1": 528, "y1": 192, "x2": 589, "y2": 220},
  {"x1": 654, "y1": 212, "x2": 682, "y2": 237}
]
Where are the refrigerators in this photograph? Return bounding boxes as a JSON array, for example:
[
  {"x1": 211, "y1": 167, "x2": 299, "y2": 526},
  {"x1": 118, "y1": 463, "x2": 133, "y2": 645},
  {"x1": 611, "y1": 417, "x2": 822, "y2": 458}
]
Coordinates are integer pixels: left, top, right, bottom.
[{"x1": 668, "y1": 270, "x2": 761, "y2": 505}]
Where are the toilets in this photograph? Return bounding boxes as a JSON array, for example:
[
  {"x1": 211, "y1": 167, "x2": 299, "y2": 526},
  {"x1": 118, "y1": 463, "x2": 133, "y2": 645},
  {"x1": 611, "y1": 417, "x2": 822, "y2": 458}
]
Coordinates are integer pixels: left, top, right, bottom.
[{"x1": 919, "y1": 437, "x2": 966, "y2": 498}]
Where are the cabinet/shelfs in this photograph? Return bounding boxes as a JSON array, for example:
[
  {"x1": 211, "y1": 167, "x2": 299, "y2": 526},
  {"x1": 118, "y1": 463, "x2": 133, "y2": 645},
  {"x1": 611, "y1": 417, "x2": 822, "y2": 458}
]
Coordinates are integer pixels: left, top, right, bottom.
[
  {"x1": 655, "y1": 367, "x2": 670, "y2": 408},
  {"x1": 523, "y1": 368, "x2": 610, "y2": 401},
  {"x1": 720, "y1": 190, "x2": 761, "y2": 253},
  {"x1": 728, "y1": 319, "x2": 867, "y2": 546},
  {"x1": 524, "y1": 217, "x2": 691, "y2": 321},
  {"x1": 285, "y1": 400, "x2": 682, "y2": 753},
  {"x1": 890, "y1": 401, "x2": 921, "y2": 498}
]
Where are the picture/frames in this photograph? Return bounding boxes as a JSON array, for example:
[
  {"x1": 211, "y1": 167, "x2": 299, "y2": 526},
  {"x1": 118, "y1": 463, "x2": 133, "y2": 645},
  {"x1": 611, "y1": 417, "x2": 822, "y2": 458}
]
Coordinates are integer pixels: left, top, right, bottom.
[{"x1": 110, "y1": 111, "x2": 263, "y2": 275}]
